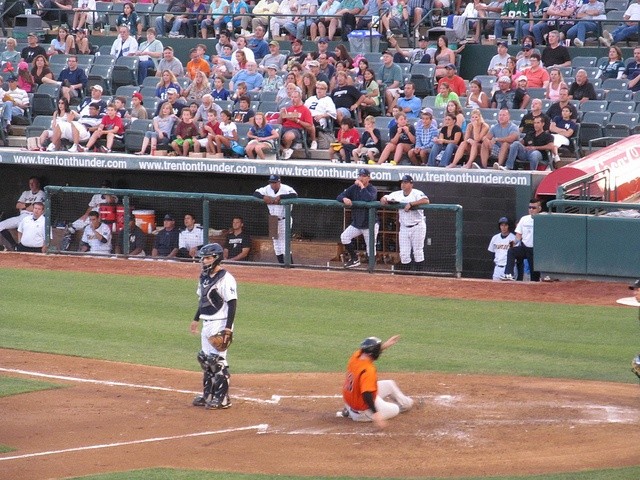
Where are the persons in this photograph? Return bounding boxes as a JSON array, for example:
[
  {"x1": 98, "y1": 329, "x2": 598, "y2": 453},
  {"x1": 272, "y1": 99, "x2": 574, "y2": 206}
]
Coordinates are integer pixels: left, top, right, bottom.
[
  {"x1": 486, "y1": 216, "x2": 522, "y2": 282},
  {"x1": 252, "y1": 174, "x2": 299, "y2": 269},
  {"x1": 0, "y1": 0, "x2": 640, "y2": 175},
  {"x1": 629, "y1": 279, "x2": 640, "y2": 378},
  {"x1": 0, "y1": 176, "x2": 46, "y2": 233},
  {"x1": 55, "y1": 184, "x2": 119, "y2": 236},
  {"x1": 499, "y1": 198, "x2": 547, "y2": 282},
  {"x1": 110, "y1": 214, "x2": 147, "y2": 260},
  {"x1": 336, "y1": 168, "x2": 381, "y2": 270},
  {"x1": 380, "y1": 174, "x2": 431, "y2": 276},
  {"x1": 17, "y1": 202, "x2": 48, "y2": 253},
  {"x1": 341, "y1": 334, "x2": 415, "y2": 422},
  {"x1": 178, "y1": 214, "x2": 229, "y2": 258},
  {"x1": 188, "y1": 242, "x2": 238, "y2": 410},
  {"x1": 151, "y1": 213, "x2": 182, "y2": 260},
  {"x1": 222, "y1": 216, "x2": 252, "y2": 265},
  {"x1": 510, "y1": 215, "x2": 531, "y2": 282},
  {"x1": 78, "y1": 211, "x2": 113, "y2": 258}
]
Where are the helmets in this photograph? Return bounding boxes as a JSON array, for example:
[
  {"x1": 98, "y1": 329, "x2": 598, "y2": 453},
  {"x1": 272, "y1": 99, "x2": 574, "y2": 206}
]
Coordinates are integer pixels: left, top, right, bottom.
[
  {"x1": 199, "y1": 242, "x2": 224, "y2": 284},
  {"x1": 361, "y1": 337, "x2": 381, "y2": 359}
]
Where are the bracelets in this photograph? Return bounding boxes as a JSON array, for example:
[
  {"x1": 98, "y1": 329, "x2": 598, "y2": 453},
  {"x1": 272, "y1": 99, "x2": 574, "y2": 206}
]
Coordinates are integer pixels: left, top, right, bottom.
[{"x1": 409, "y1": 201, "x2": 413, "y2": 208}]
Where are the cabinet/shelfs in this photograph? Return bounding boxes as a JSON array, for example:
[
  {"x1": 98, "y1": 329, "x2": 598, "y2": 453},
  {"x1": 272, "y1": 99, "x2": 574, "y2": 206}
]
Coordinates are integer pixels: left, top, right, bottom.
[{"x1": 342, "y1": 190, "x2": 400, "y2": 263}]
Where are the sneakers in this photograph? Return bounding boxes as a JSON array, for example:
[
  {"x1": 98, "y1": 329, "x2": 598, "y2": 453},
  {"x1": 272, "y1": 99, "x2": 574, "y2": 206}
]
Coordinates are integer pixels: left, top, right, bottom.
[
  {"x1": 310, "y1": 140, "x2": 318, "y2": 150},
  {"x1": 343, "y1": 256, "x2": 360, "y2": 269},
  {"x1": 390, "y1": 160, "x2": 399, "y2": 165},
  {"x1": 193, "y1": 396, "x2": 207, "y2": 406},
  {"x1": 493, "y1": 162, "x2": 509, "y2": 170},
  {"x1": 281, "y1": 147, "x2": 294, "y2": 159},
  {"x1": 599, "y1": 37, "x2": 611, "y2": 47},
  {"x1": 574, "y1": 37, "x2": 584, "y2": 47},
  {"x1": 386, "y1": 30, "x2": 395, "y2": 39},
  {"x1": 472, "y1": 161, "x2": 483, "y2": 169},
  {"x1": 416, "y1": 30, "x2": 420, "y2": 38},
  {"x1": 68, "y1": 145, "x2": 77, "y2": 153},
  {"x1": 100, "y1": 145, "x2": 110, "y2": 153},
  {"x1": 293, "y1": 143, "x2": 303, "y2": 150},
  {"x1": 206, "y1": 400, "x2": 232, "y2": 409},
  {"x1": 46, "y1": 143, "x2": 55, "y2": 152},
  {"x1": 500, "y1": 273, "x2": 515, "y2": 281},
  {"x1": 342, "y1": 408, "x2": 351, "y2": 418},
  {"x1": 603, "y1": 29, "x2": 614, "y2": 43},
  {"x1": 553, "y1": 161, "x2": 567, "y2": 169},
  {"x1": 7, "y1": 124, "x2": 12, "y2": 135},
  {"x1": 77, "y1": 144, "x2": 88, "y2": 152},
  {"x1": 367, "y1": 160, "x2": 376, "y2": 165},
  {"x1": 62, "y1": 79, "x2": 70, "y2": 86}
]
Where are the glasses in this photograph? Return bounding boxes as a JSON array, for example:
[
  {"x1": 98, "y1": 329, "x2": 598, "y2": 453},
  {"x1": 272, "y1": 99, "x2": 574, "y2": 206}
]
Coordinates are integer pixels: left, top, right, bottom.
[
  {"x1": 522, "y1": 49, "x2": 530, "y2": 52},
  {"x1": 529, "y1": 206, "x2": 538, "y2": 209}
]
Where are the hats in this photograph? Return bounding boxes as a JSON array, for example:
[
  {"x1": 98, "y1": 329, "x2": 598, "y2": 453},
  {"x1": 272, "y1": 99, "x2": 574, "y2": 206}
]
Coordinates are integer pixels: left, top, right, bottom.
[
  {"x1": 399, "y1": 175, "x2": 412, "y2": 183},
  {"x1": 420, "y1": 108, "x2": 434, "y2": 115},
  {"x1": 266, "y1": 63, "x2": 279, "y2": 71},
  {"x1": 309, "y1": 60, "x2": 320, "y2": 68},
  {"x1": 162, "y1": 214, "x2": 175, "y2": 220},
  {"x1": 317, "y1": 37, "x2": 327, "y2": 43},
  {"x1": 358, "y1": 168, "x2": 369, "y2": 177},
  {"x1": 499, "y1": 217, "x2": 509, "y2": 224},
  {"x1": 90, "y1": 85, "x2": 103, "y2": 92},
  {"x1": 166, "y1": 88, "x2": 177, "y2": 95},
  {"x1": 291, "y1": 39, "x2": 303, "y2": 45},
  {"x1": 518, "y1": 75, "x2": 527, "y2": 81},
  {"x1": 382, "y1": 50, "x2": 392, "y2": 57},
  {"x1": 522, "y1": 43, "x2": 531, "y2": 49},
  {"x1": 74, "y1": 28, "x2": 84, "y2": 33},
  {"x1": 268, "y1": 41, "x2": 279, "y2": 48},
  {"x1": 27, "y1": 32, "x2": 35, "y2": 36},
  {"x1": 163, "y1": 45, "x2": 172, "y2": 51},
  {"x1": 629, "y1": 279, "x2": 640, "y2": 290},
  {"x1": 445, "y1": 64, "x2": 457, "y2": 71},
  {"x1": 131, "y1": 93, "x2": 143, "y2": 104},
  {"x1": 101, "y1": 180, "x2": 111, "y2": 186},
  {"x1": 497, "y1": 40, "x2": 508, "y2": 47},
  {"x1": 268, "y1": 174, "x2": 280, "y2": 182},
  {"x1": 6, "y1": 74, "x2": 17, "y2": 81},
  {"x1": 18, "y1": 62, "x2": 28, "y2": 70}
]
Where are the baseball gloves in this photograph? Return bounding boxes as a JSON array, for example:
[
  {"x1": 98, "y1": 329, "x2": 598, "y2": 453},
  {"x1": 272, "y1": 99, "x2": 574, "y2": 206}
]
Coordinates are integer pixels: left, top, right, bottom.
[
  {"x1": 207, "y1": 330, "x2": 233, "y2": 351},
  {"x1": 632, "y1": 355, "x2": 640, "y2": 377}
]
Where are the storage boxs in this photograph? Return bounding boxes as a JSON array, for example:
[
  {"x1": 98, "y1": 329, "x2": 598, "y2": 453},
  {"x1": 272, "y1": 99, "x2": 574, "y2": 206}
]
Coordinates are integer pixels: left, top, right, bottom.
[{"x1": 347, "y1": 30, "x2": 384, "y2": 54}]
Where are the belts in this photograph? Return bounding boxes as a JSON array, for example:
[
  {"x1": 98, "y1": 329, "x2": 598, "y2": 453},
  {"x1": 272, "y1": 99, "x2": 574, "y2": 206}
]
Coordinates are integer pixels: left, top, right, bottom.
[{"x1": 406, "y1": 222, "x2": 419, "y2": 228}]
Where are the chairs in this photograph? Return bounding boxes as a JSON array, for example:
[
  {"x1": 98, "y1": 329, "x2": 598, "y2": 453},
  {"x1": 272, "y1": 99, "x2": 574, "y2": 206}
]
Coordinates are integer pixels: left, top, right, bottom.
[
  {"x1": 94, "y1": 2, "x2": 113, "y2": 29},
  {"x1": 66, "y1": 1, "x2": 78, "y2": 26},
  {"x1": 140, "y1": 87, "x2": 157, "y2": 97},
  {"x1": 101, "y1": 96, "x2": 113, "y2": 104},
  {"x1": 580, "y1": 112, "x2": 611, "y2": 148},
  {"x1": 626, "y1": 34, "x2": 640, "y2": 47},
  {"x1": 636, "y1": 103, "x2": 640, "y2": 113},
  {"x1": 464, "y1": 108, "x2": 473, "y2": 119},
  {"x1": 504, "y1": 27, "x2": 515, "y2": 31},
  {"x1": 471, "y1": 75, "x2": 496, "y2": 85},
  {"x1": 375, "y1": 117, "x2": 393, "y2": 128},
  {"x1": 1, "y1": 63, "x2": 18, "y2": 81},
  {"x1": 125, "y1": 120, "x2": 153, "y2": 153},
  {"x1": 323, "y1": 118, "x2": 334, "y2": 135},
  {"x1": 581, "y1": 100, "x2": 608, "y2": 111},
  {"x1": 485, "y1": 120, "x2": 497, "y2": 128},
  {"x1": 192, "y1": 3, "x2": 210, "y2": 38},
  {"x1": 49, "y1": 63, "x2": 67, "y2": 80},
  {"x1": 547, "y1": 66, "x2": 572, "y2": 77},
  {"x1": 525, "y1": 100, "x2": 552, "y2": 109},
  {"x1": 249, "y1": 91, "x2": 259, "y2": 101},
  {"x1": 633, "y1": 91, "x2": 640, "y2": 101},
  {"x1": 512, "y1": 121, "x2": 521, "y2": 126},
  {"x1": 608, "y1": 101, "x2": 636, "y2": 112},
  {"x1": 443, "y1": 0, "x2": 456, "y2": 14},
  {"x1": 77, "y1": 65, "x2": 91, "y2": 77},
  {"x1": 99, "y1": 45, "x2": 112, "y2": 55},
  {"x1": 261, "y1": 124, "x2": 282, "y2": 160},
  {"x1": 364, "y1": 52, "x2": 382, "y2": 62},
  {"x1": 235, "y1": 124, "x2": 252, "y2": 158},
  {"x1": 113, "y1": 56, "x2": 140, "y2": 85},
  {"x1": 349, "y1": 52, "x2": 363, "y2": 59},
  {"x1": 479, "y1": 108, "x2": 500, "y2": 120},
  {"x1": 509, "y1": 109, "x2": 528, "y2": 120},
  {"x1": 377, "y1": 128, "x2": 391, "y2": 150},
  {"x1": 88, "y1": 65, "x2": 114, "y2": 94},
  {"x1": 357, "y1": 128, "x2": 365, "y2": 137},
  {"x1": 116, "y1": 85, "x2": 142, "y2": 95},
  {"x1": 422, "y1": 96, "x2": 435, "y2": 107},
  {"x1": 95, "y1": 55, "x2": 116, "y2": 65},
  {"x1": 104, "y1": 2, "x2": 124, "y2": 31},
  {"x1": 76, "y1": 55, "x2": 95, "y2": 64},
  {"x1": 570, "y1": 100, "x2": 579, "y2": 110},
  {"x1": 605, "y1": 0, "x2": 629, "y2": 11},
  {"x1": 208, "y1": 79, "x2": 214, "y2": 88},
  {"x1": 410, "y1": 0, "x2": 434, "y2": 28},
  {"x1": 297, "y1": 129, "x2": 309, "y2": 158},
  {"x1": 432, "y1": 108, "x2": 445, "y2": 118},
  {"x1": 146, "y1": 4, "x2": 168, "y2": 28},
  {"x1": 33, "y1": 85, "x2": 60, "y2": 115},
  {"x1": 517, "y1": 151, "x2": 555, "y2": 171},
  {"x1": 597, "y1": 57, "x2": 623, "y2": 67},
  {"x1": 456, "y1": 55, "x2": 461, "y2": 75},
  {"x1": 435, "y1": 118, "x2": 443, "y2": 128},
  {"x1": 15, "y1": 43, "x2": 27, "y2": 52},
  {"x1": 559, "y1": 123, "x2": 580, "y2": 159},
  {"x1": 468, "y1": 86, "x2": 493, "y2": 96},
  {"x1": 27, "y1": 63, "x2": 33, "y2": 72},
  {"x1": 259, "y1": 102, "x2": 277, "y2": 112},
  {"x1": 396, "y1": 63, "x2": 411, "y2": 74},
  {"x1": 404, "y1": 74, "x2": 412, "y2": 83},
  {"x1": 177, "y1": 78, "x2": 190, "y2": 90},
  {"x1": 368, "y1": 62, "x2": 384, "y2": 74},
  {"x1": 459, "y1": 97, "x2": 466, "y2": 107},
  {"x1": 13, "y1": 93, "x2": 33, "y2": 124},
  {"x1": 142, "y1": 77, "x2": 160, "y2": 87},
  {"x1": 101, "y1": 119, "x2": 130, "y2": 147},
  {"x1": 527, "y1": 88, "x2": 546, "y2": 99},
  {"x1": 147, "y1": 108, "x2": 157, "y2": 120},
  {"x1": 574, "y1": 67, "x2": 599, "y2": 78},
  {"x1": 588, "y1": 79, "x2": 602, "y2": 89},
  {"x1": 411, "y1": 64, "x2": 436, "y2": 96},
  {"x1": 366, "y1": 83, "x2": 386, "y2": 116},
  {"x1": 390, "y1": 5, "x2": 412, "y2": 47},
  {"x1": 577, "y1": 111, "x2": 583, "y2": 122},
  {"x1": 586, "y1": 24, "x2": 600, "y2": 46},
  {"x1": 49, "y1": 54, "x2": 69, "y2": 63},
  {"x1": 351, "y1": 109, "x2": 360, "y2": 127},
  {"x1": 0, "y1": 43, "x2": 7, "y2": 51},
  {"x1": 26, "y1": 116, "x2": 53, "y2": 138},
  {"x1": 606, "y1": 90, "x2": 632, "y2": 100},
  {"x1": 572, "y1": 56, "x2": 597, "y2": 67},
  {"x1": 595, "y1": 90, "x2": 606, "y2": 100},
  {"x1": 92, "y1": 45, "x2": 99, "y2": 51},
  {"x1": 623, "y1": 58, "x2": 634, "y2": 67},
  {"x1": 259, "y1": 92, "x2": 277, "y2": 102},
  {"x1": 408, "y1": 119, "x2": 418, "y2": 129},
  {"x1": 135, "y1": 4, "x2": 154, "y2": 31},
  {"x1": 215, "y1": 100, "x2": 234, "y2": 111},
  {"x1": 603, "y1": 78, "x2": 628, "y2": 90},
  {"x1": 235, "y1": 101, "x2": 259, "y2": 112},
  {"x1": 126, "y1": 97, "x2": 132, "y2": 108},
  {"x1": 602, "y1": 11, "x2": 625, "y2": 34},
  {"x1": 630, "y1": 126, "x2": 640, "y2": 135},
  {"x1": 143, "y1": 97, "x2": 161, "y2": 108},
  {"x1": 279, "y1": 49, "x2": 290, "y2": 55},
  {"x1": 606, "y1": 113, "x2": 639, "y2": 146},
  {"x1": 39, "y1": 44, "x2": 50, "y2": 51}
]
